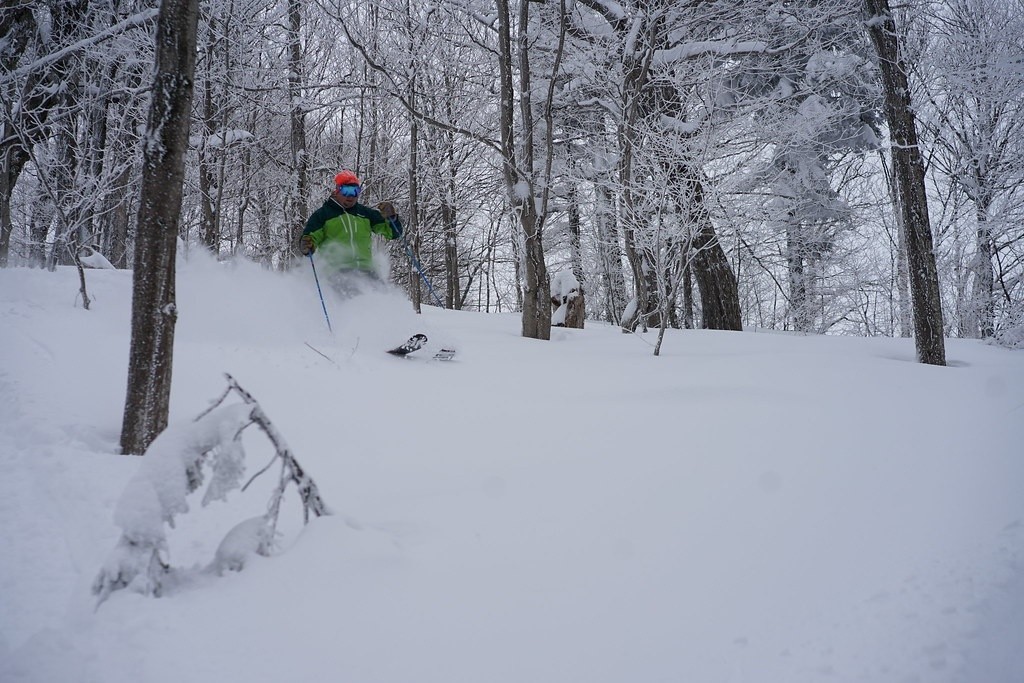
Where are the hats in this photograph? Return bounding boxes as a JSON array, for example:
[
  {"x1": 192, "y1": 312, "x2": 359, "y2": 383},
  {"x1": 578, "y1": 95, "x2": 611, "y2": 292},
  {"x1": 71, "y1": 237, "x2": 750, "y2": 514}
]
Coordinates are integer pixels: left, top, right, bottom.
[{"x1": 335, "y1": 171, "x2": 360, "y2": 193}]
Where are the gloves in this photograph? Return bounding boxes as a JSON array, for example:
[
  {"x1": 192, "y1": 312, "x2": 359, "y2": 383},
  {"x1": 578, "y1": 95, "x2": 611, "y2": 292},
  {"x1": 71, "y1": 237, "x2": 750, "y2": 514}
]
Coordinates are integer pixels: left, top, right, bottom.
[
  {"x1": 300, "y1": 236, "x2": 317, "y2": 255},
  {"x1": 378, "y1": 202, "x2": 396, "y2": 219}
]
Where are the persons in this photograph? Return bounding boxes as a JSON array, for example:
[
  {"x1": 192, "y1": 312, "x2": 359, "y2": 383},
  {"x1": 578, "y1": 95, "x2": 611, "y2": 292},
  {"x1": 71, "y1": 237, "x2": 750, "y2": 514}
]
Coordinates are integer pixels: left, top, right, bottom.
[{"x1": 298, "y1": 169, "x2": 403, "y2": 303}]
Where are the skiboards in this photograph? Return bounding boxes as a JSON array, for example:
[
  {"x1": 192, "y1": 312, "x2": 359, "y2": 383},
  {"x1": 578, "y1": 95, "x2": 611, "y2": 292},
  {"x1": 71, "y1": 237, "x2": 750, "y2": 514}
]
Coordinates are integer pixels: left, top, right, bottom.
[{"x1": 384, "y1": 333, "x2": 456, "y2": 372}]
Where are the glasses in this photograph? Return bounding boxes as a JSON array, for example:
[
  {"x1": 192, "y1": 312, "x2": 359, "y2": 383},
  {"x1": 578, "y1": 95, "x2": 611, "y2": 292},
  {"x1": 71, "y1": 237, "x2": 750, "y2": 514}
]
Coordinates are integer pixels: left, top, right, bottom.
[{"x1": 340, "y1": 185, "x2": 361, "y2": 198}]
